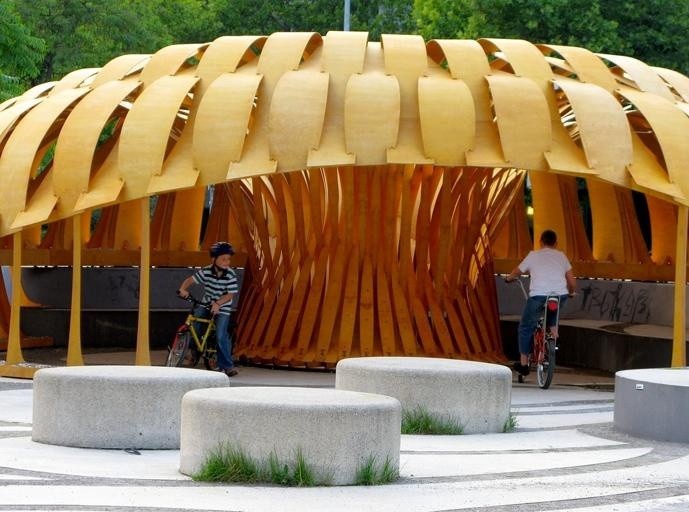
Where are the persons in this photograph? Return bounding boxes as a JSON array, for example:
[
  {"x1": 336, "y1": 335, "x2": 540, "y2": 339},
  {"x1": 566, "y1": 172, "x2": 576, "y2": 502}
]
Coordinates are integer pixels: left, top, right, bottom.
[
  {"x1": 164, "y1": 240, "x2": 240, "y2": 377},
  {"x1": 508, "y1": 230, "x2": 577, "y2": 374}
]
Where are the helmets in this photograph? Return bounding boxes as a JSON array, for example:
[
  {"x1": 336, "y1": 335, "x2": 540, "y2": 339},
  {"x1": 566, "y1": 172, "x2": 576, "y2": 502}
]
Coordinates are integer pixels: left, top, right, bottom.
[{"x1": 209, "y1": 240, "x2": 236, "y2": 257}]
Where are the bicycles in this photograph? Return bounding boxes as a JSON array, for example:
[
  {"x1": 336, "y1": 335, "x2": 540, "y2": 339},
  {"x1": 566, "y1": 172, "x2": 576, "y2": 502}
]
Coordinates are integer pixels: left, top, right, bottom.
[
  {"x1": 497, "y1": 272, "x2": 579, "y2": 387},
  {"x1": 161, "y1": 289, "x2": 241, "y2": 371}
]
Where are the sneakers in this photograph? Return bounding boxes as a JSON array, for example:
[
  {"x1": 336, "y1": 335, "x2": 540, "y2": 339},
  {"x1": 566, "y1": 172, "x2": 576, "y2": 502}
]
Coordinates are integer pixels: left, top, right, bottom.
[
  {"x1": 512, "y1": 362, "x2": 530, "y2": 376},
  {"x1": 224, "y1": 368, "x2": 237, "y2": 376}
]
